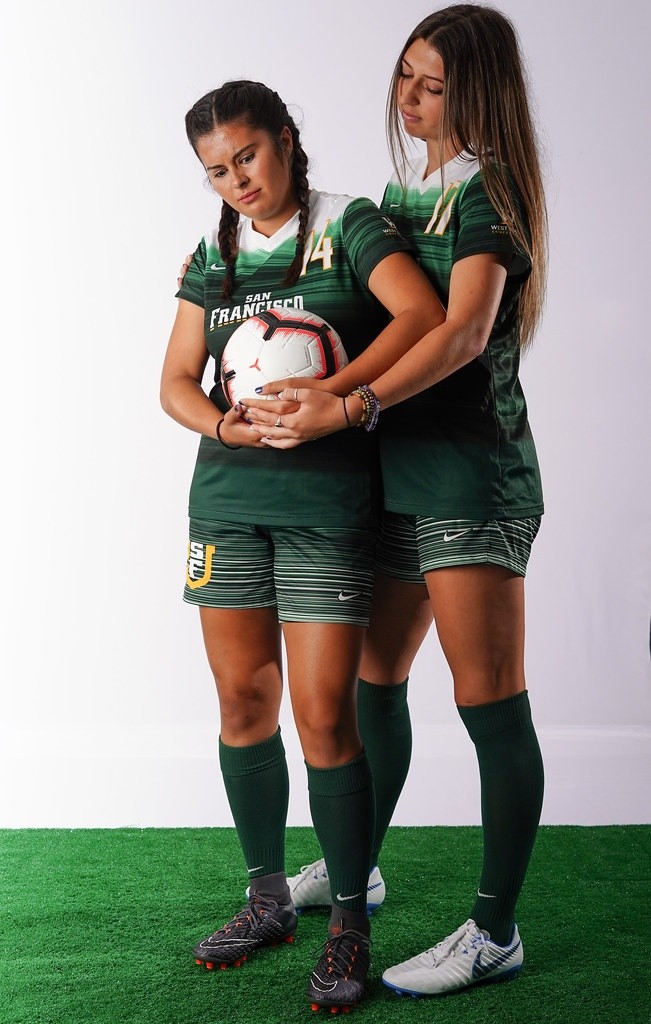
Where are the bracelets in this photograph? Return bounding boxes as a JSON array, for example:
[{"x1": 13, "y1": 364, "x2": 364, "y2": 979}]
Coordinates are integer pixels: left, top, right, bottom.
[
  {"x1": 349, "y1": 385, "x2": 379, "y2": 432},
  {"x1": 216, "y1": 418, "x2": 242, "y2": 450},
  {"x1": 342, "y1": 398, "x2": 353, "y2": 429}
]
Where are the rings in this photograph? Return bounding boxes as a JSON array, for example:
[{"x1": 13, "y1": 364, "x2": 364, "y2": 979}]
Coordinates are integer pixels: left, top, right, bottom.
[
  {"x1": 275, "y1": 416, "x2": 283, "y2": 427},
  {"x1": 294, "y1": 388, "x2": 298, "y2": 402}
]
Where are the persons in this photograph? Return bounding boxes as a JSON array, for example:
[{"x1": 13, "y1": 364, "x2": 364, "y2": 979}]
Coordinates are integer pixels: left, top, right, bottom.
[
  {"x1": 161, "y1": 79, "x2": 449, "y2": 1012},
  {"x1": 245, "y1": 4, "x2": 546, "y2": 995}
]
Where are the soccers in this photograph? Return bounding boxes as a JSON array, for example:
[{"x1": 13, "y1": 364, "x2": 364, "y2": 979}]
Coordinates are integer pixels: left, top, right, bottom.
[{"x1": 222, "y1": 307, "x2": 347, "y2": 417}]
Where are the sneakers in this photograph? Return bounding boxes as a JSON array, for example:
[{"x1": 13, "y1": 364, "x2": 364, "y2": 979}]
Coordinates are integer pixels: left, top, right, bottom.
[
  {"x1": 306, "y1": 915, "x2": 374, "y2": 1015},
  {"x1": 379, "y1": 915, "x2": 525, "y2": 1001},
  {"x1": 192, "y1": 892, "x2": 299, "y2": 971},
  {"x1": 244, "y1": 856, "x2": 386, "y2": 915}
]
